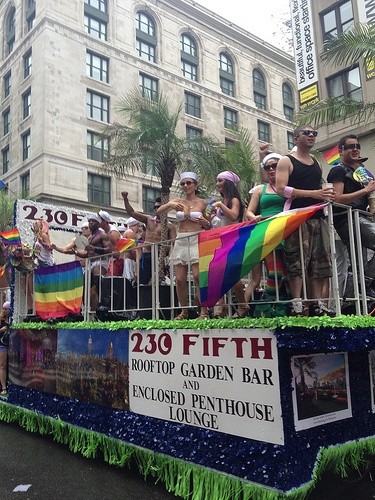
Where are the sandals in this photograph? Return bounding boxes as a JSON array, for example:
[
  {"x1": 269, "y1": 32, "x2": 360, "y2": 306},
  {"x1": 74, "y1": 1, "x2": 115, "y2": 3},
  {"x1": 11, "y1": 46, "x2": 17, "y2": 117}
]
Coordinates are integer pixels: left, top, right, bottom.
[
  {"x1": 1, "y1": 389, "x2": 7, "y2": 396},
  {"x1": 174, "y1": 309, "x2": 251, "y2": 321}
]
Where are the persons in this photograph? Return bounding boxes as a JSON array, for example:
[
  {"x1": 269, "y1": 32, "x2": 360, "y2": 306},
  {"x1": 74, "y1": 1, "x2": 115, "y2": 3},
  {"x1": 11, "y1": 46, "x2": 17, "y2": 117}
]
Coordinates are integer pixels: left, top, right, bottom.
[
  {"x1": 23, "y1": 219, "x2": 55, "y2": 322},
  {"x1": 275, "y1": 123, "x2": 337, "y2": 317},
  {"x1": 0, "y1": 301, "x2": 11, "y2": 395},
  {"x1": 327, "y1": 134, "x2": 375, "y2": 314},
  {"x1": 50, "y1": 153, "x2": 375, "y2": 320}
]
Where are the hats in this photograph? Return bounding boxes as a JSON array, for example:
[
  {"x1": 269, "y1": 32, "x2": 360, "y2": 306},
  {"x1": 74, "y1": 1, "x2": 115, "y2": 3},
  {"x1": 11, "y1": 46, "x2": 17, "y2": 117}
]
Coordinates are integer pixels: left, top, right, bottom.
[
  {"x1": 87, "y1": 213, "x2": 104, "y2": 224},
  {"x1": 98, "y1": 210, "x2": 112, "y2": 222},
  {"x1": 263, "y1": 153, "x2": 285, "y2": 165},
  {"x1": 2, "y1": 301, "x2": 11, "y2": 308},
  {"x1": 180, "y1": 172, "x2": 200, "y2": 183},
  {"x1": 126, "y1": 217, "x2": 139, "y2": 226},
  {"x1": 216, "y1": 171, "x2": 240, "y2": 187}
]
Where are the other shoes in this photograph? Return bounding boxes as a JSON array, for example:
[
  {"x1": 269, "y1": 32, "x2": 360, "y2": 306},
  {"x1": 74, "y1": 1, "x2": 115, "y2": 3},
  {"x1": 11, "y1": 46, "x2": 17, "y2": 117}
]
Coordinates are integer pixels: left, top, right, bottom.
[
  {"x1": 313, "y1": 300, "x2": 337, "y2": 318},
  {"x1": 290, "y1": 299, "x2": 310, "y2": 317}
]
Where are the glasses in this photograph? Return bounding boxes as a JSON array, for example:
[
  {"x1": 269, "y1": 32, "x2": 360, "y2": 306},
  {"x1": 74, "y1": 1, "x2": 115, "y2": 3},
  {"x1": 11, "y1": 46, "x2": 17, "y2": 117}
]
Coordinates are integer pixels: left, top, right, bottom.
[
  {"x1": 264, "y1": 164, "x2": 276, "y2": 171},
  {"x1": 302, "y1": 130, "x2": 320, "y2": 137},
  {"x1": 341, "y1": 143, "x2": 362, "y2": 150},
  {"x1": 180, "y1": 181, "x2": 196, "y2": 186},
  {"x1": 81, "y1": 226, "x2": 88, "y2": 230}
]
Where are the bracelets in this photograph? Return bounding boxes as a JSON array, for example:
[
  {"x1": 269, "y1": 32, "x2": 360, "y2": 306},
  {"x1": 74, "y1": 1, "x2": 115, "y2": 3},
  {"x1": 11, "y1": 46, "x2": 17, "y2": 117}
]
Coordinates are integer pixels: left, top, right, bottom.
[
  {"x1": 74, "y1": 250, "x2": 78, "y2": 254},
  {"x1": 204, "y1": 221, "x2": 210, "y2": 229},
  {"x1": 283, "y1": 186, "x2": 295, "y2": 211}
]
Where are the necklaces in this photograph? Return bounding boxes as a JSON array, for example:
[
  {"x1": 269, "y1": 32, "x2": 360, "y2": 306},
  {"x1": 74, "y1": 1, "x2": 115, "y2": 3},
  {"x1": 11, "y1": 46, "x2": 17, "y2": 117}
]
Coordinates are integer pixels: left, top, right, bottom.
[{"x1": 270, "y1": 183, "x2": 277, "y2": 193}]
